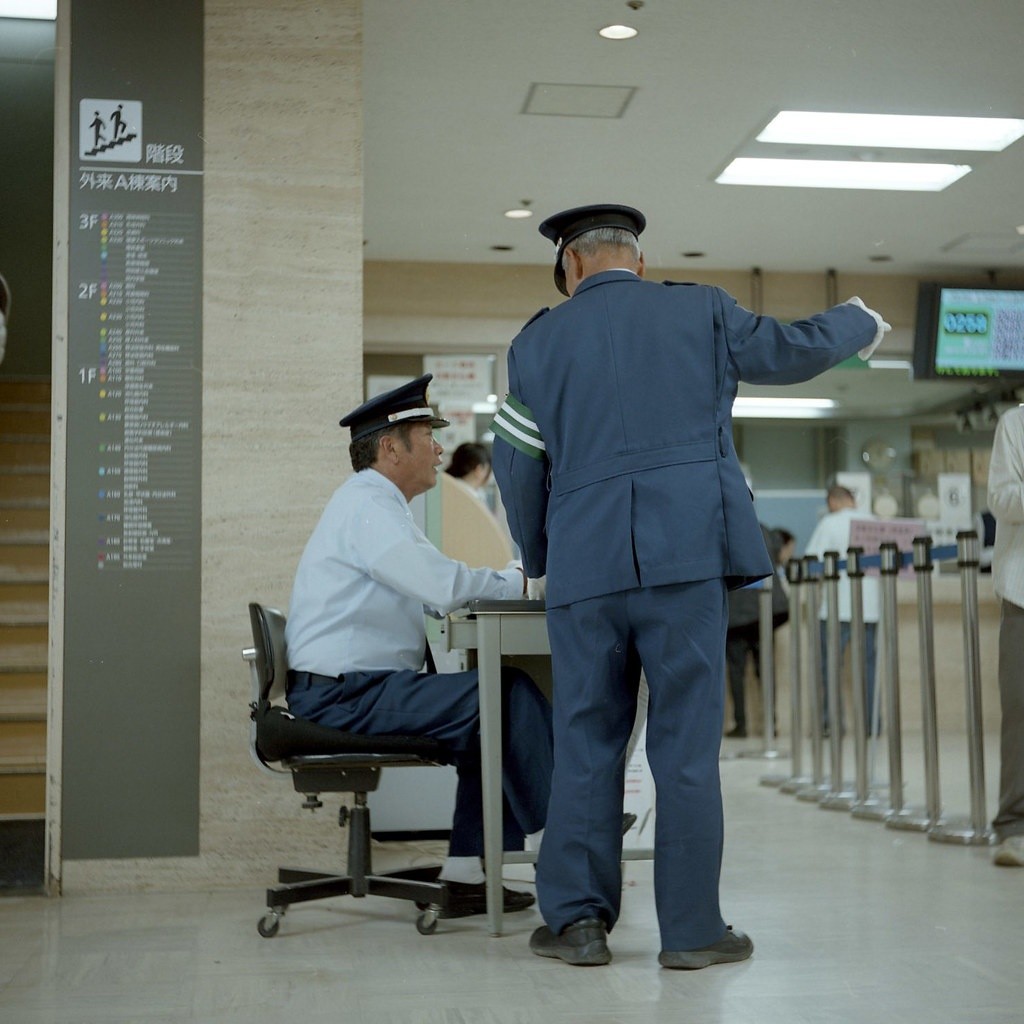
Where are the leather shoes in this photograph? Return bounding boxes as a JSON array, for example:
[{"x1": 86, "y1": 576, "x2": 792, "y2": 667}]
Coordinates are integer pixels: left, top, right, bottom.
[
  {"x1": 531, "y1": 917, "x2": 612, "y2": 965},
  {"x1": 434, "y1": 877, "x2": 535, "y2": 918},
  {"x1": 533, "y1": 813, "x2": 637, "y2": 870},
  {"x1": 659, "y1": 925, "x2": 754, "y2": 969}
]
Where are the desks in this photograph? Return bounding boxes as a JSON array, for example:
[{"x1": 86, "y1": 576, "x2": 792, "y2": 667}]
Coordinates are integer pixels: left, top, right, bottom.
[{"x1": 442, "y1": 599, "x2": 655, "y2": 936}]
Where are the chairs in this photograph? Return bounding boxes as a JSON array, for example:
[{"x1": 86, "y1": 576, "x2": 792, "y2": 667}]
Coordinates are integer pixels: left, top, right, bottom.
[{"x1": 241, "y1": 602, "x2": 445, "y2": 937}]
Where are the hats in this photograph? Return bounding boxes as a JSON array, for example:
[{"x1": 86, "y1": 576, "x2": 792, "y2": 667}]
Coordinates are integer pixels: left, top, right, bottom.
[
  {"x1": 538, "y1": 205, "x2": 647, "y2": 296},
  {"x1": 340, "y1": 374, "x2": 450, "y2": 442}
]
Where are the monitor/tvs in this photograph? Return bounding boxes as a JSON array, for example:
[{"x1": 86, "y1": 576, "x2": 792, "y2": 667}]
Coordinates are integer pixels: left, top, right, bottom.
[{"x1": 912, "y1": 280, "x2": 1024, "y2": 379}]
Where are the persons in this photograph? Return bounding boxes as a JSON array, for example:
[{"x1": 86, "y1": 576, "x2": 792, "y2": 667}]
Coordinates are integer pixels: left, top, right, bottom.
[
  {"x1": 286, "y1": 371, "x2": 637, "y2": 919},
  {"x1": 441, "y1": 441, "x2": 494, "y2": 504},
  {"x1": 490, "y1": 201, "x2": 893, "y2": 970},
  {"x1": 986, "y1": 404, "x2": 1024, "y2": 868},
  {"x1": 717, "y1": 483, "x2": 885, "y2": 740}
]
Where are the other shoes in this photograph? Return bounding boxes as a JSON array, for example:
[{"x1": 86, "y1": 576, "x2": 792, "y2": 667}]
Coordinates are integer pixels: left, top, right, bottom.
[
  {"x1": 996, "y1": 835, "x2": 1024, "y2": 865},
  {"x1": 727, "y1": 726, "x2": 746, "y2": 737}
]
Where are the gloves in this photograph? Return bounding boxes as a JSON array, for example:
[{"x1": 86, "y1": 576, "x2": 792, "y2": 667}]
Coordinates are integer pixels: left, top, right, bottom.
[
  {"x1": 526, "y1": 575, "x2": 547, "y2": 600},
  {"x1": 506, "y1": 559, "x2": 523, "y2": 572},
  {"x1": 847, "y1": 295, "x2": 892, "y2": 361}
]
